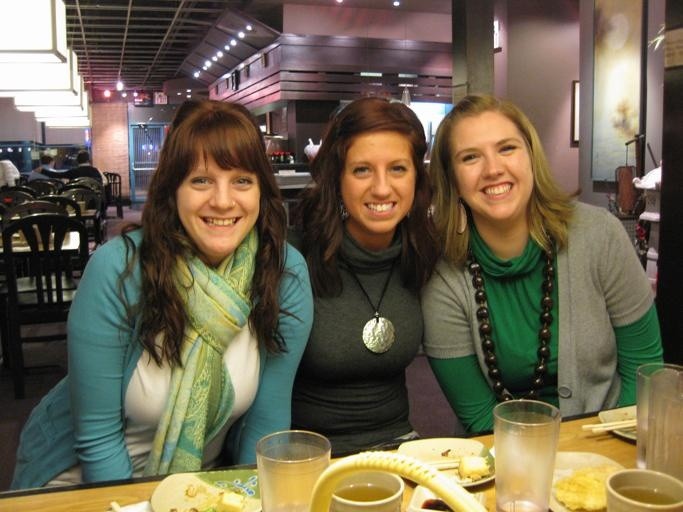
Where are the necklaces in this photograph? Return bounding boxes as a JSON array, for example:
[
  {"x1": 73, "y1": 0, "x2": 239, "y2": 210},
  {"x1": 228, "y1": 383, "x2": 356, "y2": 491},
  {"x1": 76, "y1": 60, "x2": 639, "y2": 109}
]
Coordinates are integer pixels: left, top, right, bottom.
[
  {"x1": 467, "y1": 227, "x2": 555, "y2": 409},
  {"x1": 341, "y1": 250, "x2": 401, "y2": 353}
]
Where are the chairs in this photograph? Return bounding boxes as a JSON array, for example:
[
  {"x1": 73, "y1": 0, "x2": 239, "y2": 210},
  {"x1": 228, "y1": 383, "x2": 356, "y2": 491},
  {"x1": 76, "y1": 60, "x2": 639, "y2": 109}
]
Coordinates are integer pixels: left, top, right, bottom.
[
  {"x1": 61, "y1": 189, "x2": 107, "y2": 245},
  {"x1": 4, "y1": 216, "x2": 91, "y2": 400},
  {"x1": 4, "y1": 202, "x2": 67, "y2": 225},
  {"x1": 25, "y1": 181, "x2": 58, "y2": 196},
  {"x1": 105, "y1": 174, "x2": 123, "y2": 218},
  {"x1": 61, "y1": 178, "x2": 104, "y2": 204},
  {"x1": 2, "y1": 186, "x2": 37, "y2": 198},
  {"x1": 0, "y1": 192, "x2": 35, "y2": 218},
  {"x1": 44, "y1": 179, "x2": 64, "y2": 192},
  {"x1": 38, "y1": 196, "x2": 80, "y2": 216}
]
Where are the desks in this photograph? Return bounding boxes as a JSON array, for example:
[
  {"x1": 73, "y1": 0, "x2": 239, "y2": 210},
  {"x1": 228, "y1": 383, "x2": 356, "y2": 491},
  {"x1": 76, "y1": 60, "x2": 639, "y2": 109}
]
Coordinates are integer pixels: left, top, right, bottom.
[{"x1": 0, "y1": 229, "x2": 80, "y2": 279}]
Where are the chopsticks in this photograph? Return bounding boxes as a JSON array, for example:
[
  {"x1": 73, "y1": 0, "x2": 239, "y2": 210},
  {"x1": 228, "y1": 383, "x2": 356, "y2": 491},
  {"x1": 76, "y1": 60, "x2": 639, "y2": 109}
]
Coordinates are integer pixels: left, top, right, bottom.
[{"x1": 582, "y1": 419, "x2": 637, "y2": 435}]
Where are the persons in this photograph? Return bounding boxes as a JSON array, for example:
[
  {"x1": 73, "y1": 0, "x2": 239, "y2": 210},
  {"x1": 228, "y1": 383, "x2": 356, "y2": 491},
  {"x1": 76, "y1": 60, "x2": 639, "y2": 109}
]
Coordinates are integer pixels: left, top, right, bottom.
[
  {"x1": 289, "y1": 98, "x2": 441, "y2": 457},
  {"x1": 34, "y1": 150, "x2": 108, "y2": 210},
  {"x1": 422, "y1": 94, "x2": 663, "y2": 437},
  {"x1": 9, "y1": 98, "x2": 315, "y2": 491},
  {"x1": 0, "y1": 150, "x2": 22, "y2": 218},
  {"x1": 28, "y1": 155, "x2": 55, "y2": 182}
]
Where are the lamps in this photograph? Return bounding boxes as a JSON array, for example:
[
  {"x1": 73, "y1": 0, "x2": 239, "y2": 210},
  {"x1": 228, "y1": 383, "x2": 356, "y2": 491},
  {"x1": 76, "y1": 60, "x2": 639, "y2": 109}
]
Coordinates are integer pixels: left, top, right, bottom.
[{"x1": 400, "y1": 10, "x2": 411, "y2": 105}]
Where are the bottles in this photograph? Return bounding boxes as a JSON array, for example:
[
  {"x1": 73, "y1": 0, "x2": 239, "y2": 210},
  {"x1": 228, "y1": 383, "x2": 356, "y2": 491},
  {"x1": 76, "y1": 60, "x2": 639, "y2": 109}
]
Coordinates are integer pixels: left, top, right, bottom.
[{"x1": 267, "y1": 153, "x2": 292, "y2": 163}]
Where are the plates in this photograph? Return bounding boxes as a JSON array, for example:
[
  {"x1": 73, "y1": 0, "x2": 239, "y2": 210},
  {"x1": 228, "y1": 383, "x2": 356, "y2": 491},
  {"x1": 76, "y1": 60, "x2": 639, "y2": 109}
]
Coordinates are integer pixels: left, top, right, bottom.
[
  {"x1": 598, "y1": 405, "x2": 637, "y2": 441},
  {"x1": 396, "y1": 437, "x2": 485, "y2": 471},
  {"x1": 453, "y1": 445, "x2": 496, "y2": 487},
  {"x1": 406, "y1": 483, "x2": 476, "y2": 512},
  {"x1": 548, "y1": 451, "x2": 627, "y2": 512}
]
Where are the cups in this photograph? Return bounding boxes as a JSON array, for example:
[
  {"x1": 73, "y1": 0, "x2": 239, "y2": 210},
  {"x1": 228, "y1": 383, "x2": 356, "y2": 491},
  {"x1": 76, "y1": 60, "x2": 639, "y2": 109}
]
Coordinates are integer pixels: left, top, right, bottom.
[
  {"x1": 493, "y1": 401, "x2": 561, "y2": 512},
  {"x1": 636, "y1": 361, "x2": 683, "y2": 483},
  {"x1": 605, "y1": 468, "x2": 682, "y2": 511},
  {"x1": 332, "y1": 471, "x2": 404, "y2": 512},
  {"x1": 255, "y1": 430, "x2": 332, "y2": 512}
]
